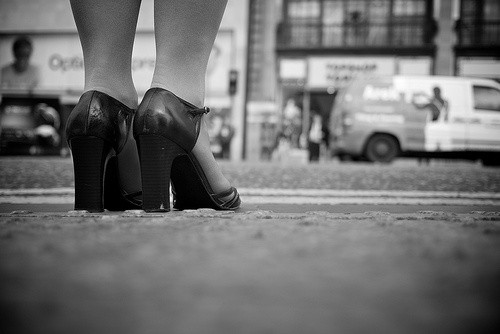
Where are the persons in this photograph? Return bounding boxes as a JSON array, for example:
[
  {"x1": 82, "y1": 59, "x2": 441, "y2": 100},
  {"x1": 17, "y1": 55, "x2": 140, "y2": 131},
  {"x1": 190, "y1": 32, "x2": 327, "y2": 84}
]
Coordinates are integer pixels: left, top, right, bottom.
[
  {"x1": 1, "y1": 35, "x2": 42, "y2": 93},
  {"x1": 307, "y1": 114, "x2": 323, "y2": 163},
  {"x1": 65, "y1": 0, "x2": 241, "y2": 212}
]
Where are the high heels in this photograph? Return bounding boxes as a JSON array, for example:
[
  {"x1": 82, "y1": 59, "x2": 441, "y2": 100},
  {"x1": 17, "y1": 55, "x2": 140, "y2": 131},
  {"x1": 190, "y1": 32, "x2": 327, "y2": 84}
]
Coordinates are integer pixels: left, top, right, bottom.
[{"x1": 64, "y1": 87, "x2": 241, "y2": 213}]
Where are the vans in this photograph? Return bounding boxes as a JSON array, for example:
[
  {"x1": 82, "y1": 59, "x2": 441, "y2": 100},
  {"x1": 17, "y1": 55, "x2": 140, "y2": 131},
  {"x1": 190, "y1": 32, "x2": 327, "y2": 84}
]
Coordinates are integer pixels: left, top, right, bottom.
[{"x1": 326, "y1": 73, "x2": 500, "y2": 164}]
